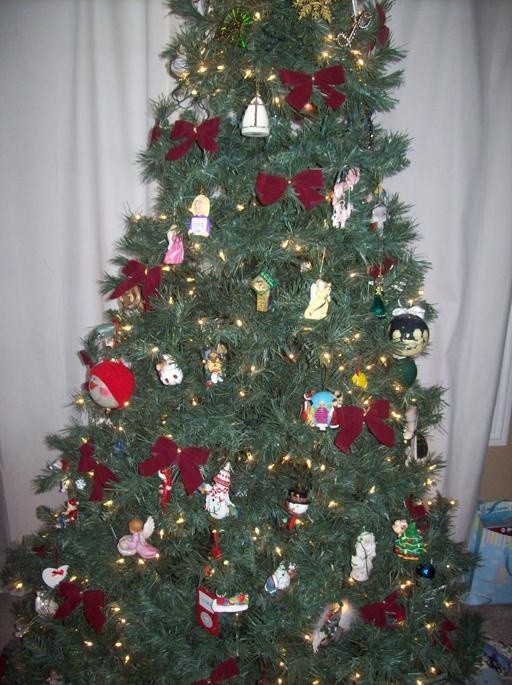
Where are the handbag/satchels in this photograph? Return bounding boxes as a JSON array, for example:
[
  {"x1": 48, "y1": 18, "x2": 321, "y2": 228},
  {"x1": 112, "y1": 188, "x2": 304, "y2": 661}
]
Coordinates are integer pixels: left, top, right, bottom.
[{"x1": 458, "y1": 499, "x2": 512, "y2": 605}]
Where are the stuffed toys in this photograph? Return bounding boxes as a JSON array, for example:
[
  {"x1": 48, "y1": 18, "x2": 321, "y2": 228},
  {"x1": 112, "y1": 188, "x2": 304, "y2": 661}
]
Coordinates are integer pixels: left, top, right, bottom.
[{"x1": 89, "y1": 359, "x2": 134, "y2": 409}]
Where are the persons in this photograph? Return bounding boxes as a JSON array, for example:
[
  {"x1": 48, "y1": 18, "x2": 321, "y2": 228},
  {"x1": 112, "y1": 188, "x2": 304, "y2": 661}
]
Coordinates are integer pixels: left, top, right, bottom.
[{"x1": 121, "y1": 519, "x2": 144, "y2": 552}]
